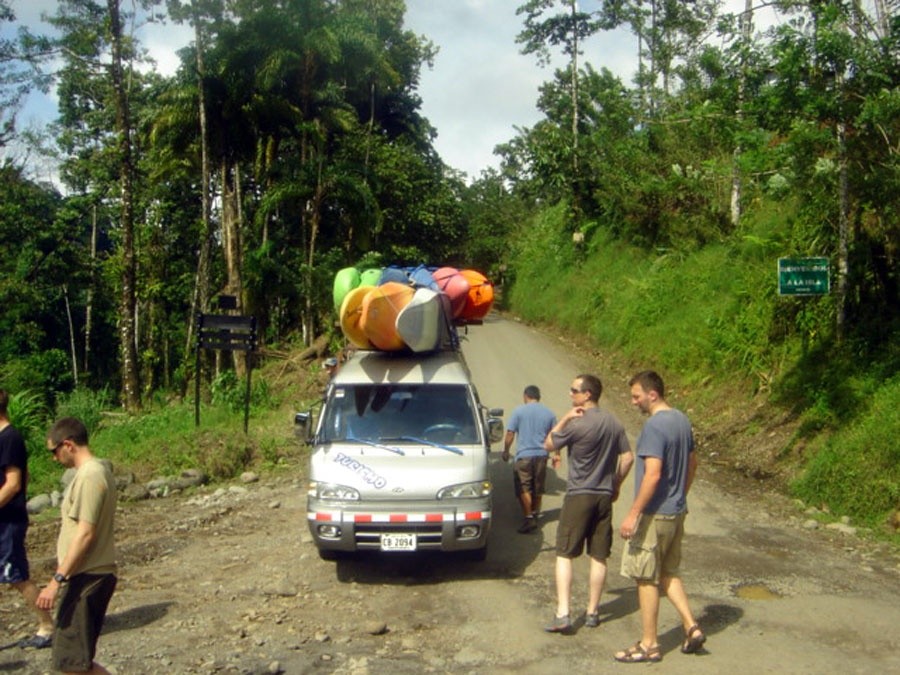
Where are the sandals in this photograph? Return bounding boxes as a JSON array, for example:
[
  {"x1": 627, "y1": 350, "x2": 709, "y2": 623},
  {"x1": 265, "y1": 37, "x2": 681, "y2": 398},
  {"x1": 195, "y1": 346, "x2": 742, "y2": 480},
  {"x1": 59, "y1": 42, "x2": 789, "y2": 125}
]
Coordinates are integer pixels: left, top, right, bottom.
[
  {"x1": 682, "y1": 625, "x2": 707, "y2": 651},
  {"x1": 612, "y1": 640, "x2": 662, "y2": 663}
]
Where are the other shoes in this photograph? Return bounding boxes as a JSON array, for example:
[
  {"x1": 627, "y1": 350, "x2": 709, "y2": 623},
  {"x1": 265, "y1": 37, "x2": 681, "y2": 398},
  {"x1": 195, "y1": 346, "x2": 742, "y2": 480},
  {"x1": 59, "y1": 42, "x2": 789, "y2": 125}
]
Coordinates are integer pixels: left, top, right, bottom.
[
  {"x1": 517, "y1": 516, "x2": 538, "y2": 533},
  {"x1": 544, "y1": 614, "x2": 572, "y2": 632},
  {"x1": 579, "y1": 610, "x2": 602, "y2": 628},
  {"x1": 533, "y1": 512, "x2": 540, "y2": 519},
  {"x1": 17, "y1": 633, "x2": 54, "y2": 652}
]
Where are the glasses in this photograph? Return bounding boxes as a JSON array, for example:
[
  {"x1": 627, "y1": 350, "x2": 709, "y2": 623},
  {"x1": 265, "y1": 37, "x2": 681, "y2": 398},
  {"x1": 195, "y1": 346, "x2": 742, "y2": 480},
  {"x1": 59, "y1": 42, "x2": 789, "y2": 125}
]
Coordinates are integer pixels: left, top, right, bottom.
[
  {"x1": 569, "y1": 386, "x2": 588, "y2": 395},
  {"x1": 50, "y1": 434, "x2": 75, "y2": 454}
]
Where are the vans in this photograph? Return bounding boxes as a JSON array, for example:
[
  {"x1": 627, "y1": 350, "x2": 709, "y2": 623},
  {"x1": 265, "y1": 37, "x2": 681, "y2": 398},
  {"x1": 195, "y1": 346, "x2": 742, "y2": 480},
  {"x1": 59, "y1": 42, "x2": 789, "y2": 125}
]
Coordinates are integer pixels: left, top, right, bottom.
[{"x1": 294, "y1": 344, "x2": 504, "y2": 563}]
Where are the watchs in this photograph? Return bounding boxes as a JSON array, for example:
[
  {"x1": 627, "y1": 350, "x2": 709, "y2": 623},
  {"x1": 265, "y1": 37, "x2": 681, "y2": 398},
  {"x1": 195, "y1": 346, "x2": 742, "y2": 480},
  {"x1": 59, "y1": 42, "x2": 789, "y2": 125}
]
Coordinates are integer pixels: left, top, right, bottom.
[{"x1": 52, "y1": 572, "x2": 67, "y2": 582}]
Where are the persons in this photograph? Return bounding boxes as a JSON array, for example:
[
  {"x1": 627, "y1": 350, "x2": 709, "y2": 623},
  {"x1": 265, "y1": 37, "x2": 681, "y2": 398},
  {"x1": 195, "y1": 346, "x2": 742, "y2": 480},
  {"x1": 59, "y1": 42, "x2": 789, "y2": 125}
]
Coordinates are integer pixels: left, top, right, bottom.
[
  {"x1": 539, "y1": 374, "x2": 635, "y2": 633},
  {"x1": 502, "y1": 384, "x2": 560, "y2": 532},
  {"x1": 0, "y1": 387, "x2": 59, "y2": 649},
  {"x1": 615, "y1": 370, "x2": 706, "y2": 665},
  {"x1": 36, "y1": 417, "x2": 120, "y2": 675}
]
